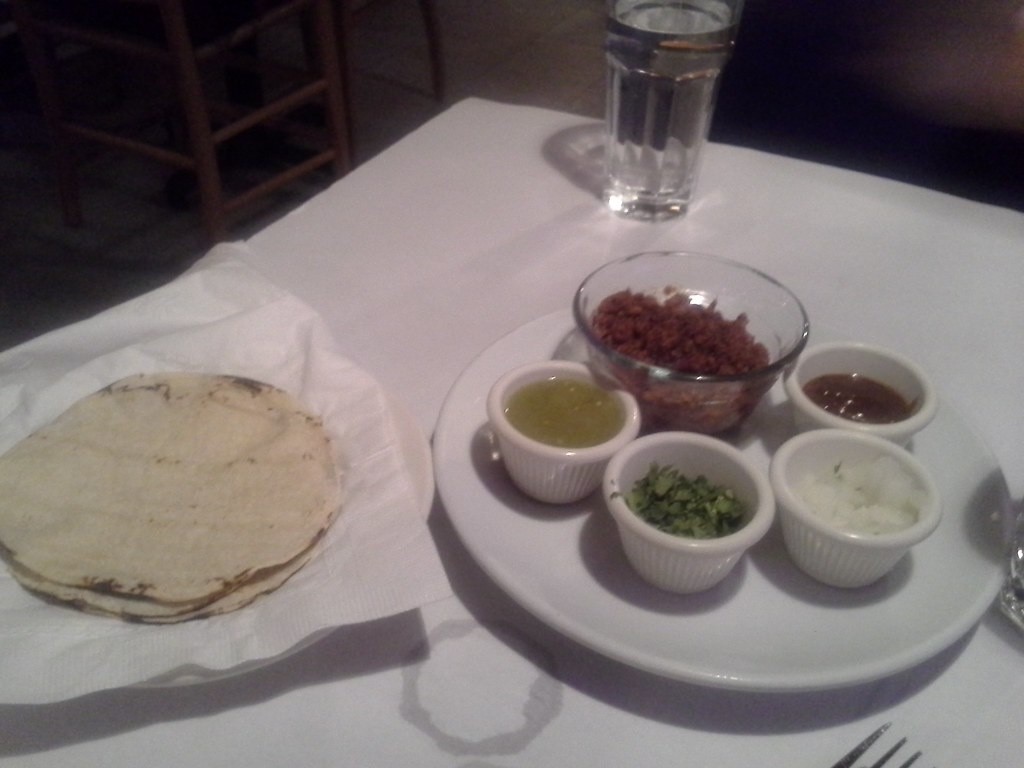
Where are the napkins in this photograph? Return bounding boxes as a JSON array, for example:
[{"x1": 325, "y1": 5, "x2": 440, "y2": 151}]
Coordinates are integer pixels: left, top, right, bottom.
[{"x1": 0, "y1": 239, "x2": 455, "y2": 710}]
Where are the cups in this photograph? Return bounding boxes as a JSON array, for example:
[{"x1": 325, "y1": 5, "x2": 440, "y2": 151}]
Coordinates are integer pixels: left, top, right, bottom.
[{"x1": 601, "y1": 0, "x2": 744, "y2": 221}]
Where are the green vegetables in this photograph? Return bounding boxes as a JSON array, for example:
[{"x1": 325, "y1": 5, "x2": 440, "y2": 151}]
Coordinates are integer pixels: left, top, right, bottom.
[{"x1": 624, "y1": 462, "x2": 744, "y2": 540}]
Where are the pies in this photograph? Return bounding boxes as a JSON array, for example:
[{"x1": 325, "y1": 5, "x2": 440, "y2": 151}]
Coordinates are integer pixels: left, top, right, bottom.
[{"x1": 0, "y1": 372, "x2": 342, "y2": 625}]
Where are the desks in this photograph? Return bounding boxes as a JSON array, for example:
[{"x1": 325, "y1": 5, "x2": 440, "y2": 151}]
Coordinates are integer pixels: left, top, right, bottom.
[{"x1": 0, "y1": 94, "x2": 1024, "y2": 768}]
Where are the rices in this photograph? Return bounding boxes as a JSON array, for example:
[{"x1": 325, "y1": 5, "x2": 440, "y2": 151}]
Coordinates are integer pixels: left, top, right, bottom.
[{"x1": 590, "y1": 284, "x2": 776, "y2": 436}]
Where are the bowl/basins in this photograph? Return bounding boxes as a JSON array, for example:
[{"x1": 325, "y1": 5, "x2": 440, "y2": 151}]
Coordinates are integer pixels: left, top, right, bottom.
[
  {"x1": 783, "y1": 341, "x2": 937, "y2": 453},
  {"x1": 605, "y1": 431, "x2": 776, "y2": 596},
  {"x1": 573, "y1": 252, "x2": 810, "y2": 438},
  {"x1": 768, "y1": 429, "x2": 943, "y2": 589},
  {"x1": 487, "y1": 360, "x2": 641, "y2": 503}
]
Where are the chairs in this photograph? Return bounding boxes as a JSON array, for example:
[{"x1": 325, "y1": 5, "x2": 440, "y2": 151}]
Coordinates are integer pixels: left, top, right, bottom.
[{"x1": 6, "y1": 0, "x2": 447, "y2": 250}]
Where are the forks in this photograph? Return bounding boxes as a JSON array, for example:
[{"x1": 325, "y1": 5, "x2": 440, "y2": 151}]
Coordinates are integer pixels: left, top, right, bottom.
[{"x1": 831, "y1": 721, "x2": 919, "y2": 768}]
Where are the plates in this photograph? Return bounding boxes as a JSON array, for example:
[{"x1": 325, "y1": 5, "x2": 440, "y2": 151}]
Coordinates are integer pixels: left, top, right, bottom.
[
  {"x1": 433, "y1": 310, "x2": 1012, "y2": 692},
  {"x1": 120, "y1": 392, "x2": 440, "y2": 688}
]
